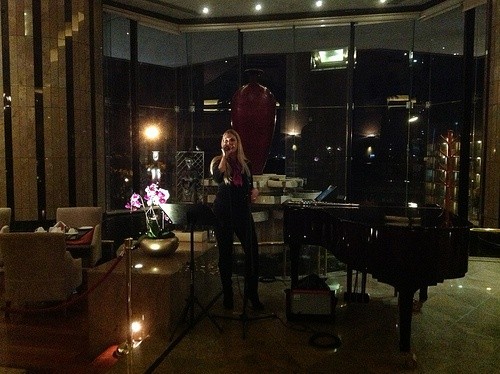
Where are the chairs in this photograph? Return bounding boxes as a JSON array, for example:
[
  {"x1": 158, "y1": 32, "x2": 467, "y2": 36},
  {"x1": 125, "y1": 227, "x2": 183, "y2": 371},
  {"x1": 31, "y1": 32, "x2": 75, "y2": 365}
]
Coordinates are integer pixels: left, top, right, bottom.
[{"x1": 0, "y1": 205, "x2": 102, "y2": 320}]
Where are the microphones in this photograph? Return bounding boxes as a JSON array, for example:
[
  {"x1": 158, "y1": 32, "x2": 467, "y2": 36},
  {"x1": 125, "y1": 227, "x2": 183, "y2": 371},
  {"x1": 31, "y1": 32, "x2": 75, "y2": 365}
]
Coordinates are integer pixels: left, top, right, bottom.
[{"x1": 224, "y1": 145, "x2": 233, "y2": 151}]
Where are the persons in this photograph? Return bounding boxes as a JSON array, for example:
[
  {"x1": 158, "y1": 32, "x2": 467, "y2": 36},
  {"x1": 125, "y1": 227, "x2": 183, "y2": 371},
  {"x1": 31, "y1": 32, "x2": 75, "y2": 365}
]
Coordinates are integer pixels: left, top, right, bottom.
[{"x1": 209, "y1": 129, "x2": 264, "y2": 313}]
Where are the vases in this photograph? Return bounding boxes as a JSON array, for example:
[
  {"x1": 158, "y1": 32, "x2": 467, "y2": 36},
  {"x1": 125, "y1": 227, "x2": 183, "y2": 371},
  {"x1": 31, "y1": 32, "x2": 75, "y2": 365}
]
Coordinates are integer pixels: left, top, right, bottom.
[
  {"x1": 230, "y1": 69, "x2": 277, "y2": 176},
  {"x1": 141, "y1": 238, "x2": 179, "y2": 257}
]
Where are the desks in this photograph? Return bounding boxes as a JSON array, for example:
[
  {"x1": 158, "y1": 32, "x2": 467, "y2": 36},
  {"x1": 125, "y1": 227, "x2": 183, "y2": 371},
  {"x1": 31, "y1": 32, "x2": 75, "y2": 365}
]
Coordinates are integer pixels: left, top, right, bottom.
[{"x1": 65, "y1": 229, "x2": 90, "y2": 240}]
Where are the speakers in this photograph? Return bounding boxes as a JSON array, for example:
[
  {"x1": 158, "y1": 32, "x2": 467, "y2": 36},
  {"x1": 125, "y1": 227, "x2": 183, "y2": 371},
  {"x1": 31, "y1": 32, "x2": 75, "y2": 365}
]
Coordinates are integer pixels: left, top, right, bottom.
[{"x1": 290, "y1": 290, "x2": 332, "y2": 317}]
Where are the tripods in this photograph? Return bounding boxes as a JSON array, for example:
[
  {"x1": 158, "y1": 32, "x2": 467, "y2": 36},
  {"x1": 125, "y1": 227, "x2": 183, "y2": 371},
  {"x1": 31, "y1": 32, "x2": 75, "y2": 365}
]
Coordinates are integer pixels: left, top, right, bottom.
[
  {"x1": 167, "y1": 205, "x2": 224, "y2": 340},
  {"x1": 211, "y1": 153, "x2": 275, "y2": 339}
]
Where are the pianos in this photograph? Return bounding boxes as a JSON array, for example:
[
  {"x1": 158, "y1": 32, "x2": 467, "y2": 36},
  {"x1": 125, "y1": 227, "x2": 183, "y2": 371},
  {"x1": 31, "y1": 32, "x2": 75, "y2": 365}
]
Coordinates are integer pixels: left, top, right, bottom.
[{"x1": 282, "y1": 198, "x2": 468, "y2": 354}]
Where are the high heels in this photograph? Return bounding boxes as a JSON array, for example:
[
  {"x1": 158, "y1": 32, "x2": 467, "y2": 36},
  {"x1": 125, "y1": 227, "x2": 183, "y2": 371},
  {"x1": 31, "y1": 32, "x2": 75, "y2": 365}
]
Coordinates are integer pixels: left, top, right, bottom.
[
  {"x1": 243, "y1": 289, "x2": 266, "y2": 313},
  {"x1": 223, "y1": 290, "x2": 234, "y2": 309}
]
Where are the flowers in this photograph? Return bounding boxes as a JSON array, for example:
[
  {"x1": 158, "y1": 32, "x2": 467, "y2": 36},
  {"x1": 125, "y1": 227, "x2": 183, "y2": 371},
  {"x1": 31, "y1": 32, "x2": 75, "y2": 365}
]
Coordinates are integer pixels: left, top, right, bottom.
[{"x1": 125, "y1": 184, "x2": 177, "y2": 240}]
